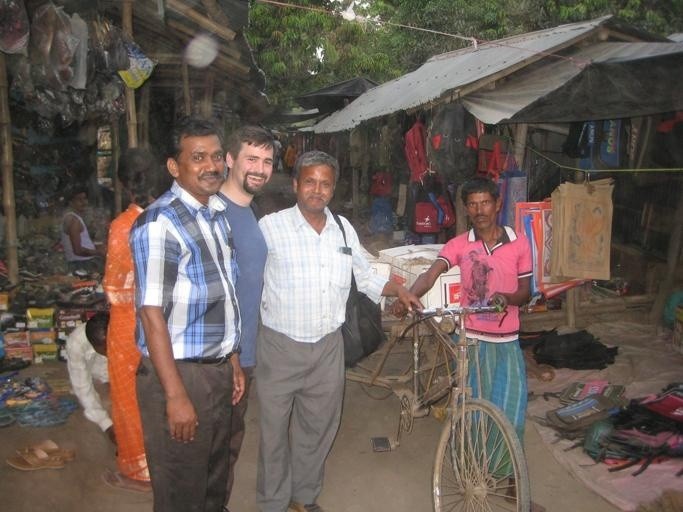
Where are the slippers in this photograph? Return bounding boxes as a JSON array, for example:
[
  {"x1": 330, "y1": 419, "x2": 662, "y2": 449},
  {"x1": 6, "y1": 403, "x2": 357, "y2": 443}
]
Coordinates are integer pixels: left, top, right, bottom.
[
  {"x1": 102, "y1": 466, "x2": 154, "y2": 495},
  {"x1": 6, "y1": 447, "x2": 68, "y2": 472},
  {"x1": 15, "y1": 439, "x2": 76, "y2": 461},
  {"x1": 0, "y1": 375, "x2": 82, "y2": 432}
]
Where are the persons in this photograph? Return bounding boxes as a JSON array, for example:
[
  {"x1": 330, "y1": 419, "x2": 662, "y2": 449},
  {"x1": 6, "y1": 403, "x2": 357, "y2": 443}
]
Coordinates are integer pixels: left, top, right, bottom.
[
  {"x1": 127, "y1": 114, "x2": 246, "y2": 512},
  {"x1": 252, "y1": 148, "x2": 426, "y2": 512},
  {"x1": 57, "y1": 185, "x2": 104, "y2": 281},
  {"x1": 215, "y1": 125, "x2": 279, "y2": 512},
  {"x1": 100, "y1": 144, "x2": 166, "y2": 493},
  {"x1": 64, "y1": 310, "x2": 117, "y2": 450},
  {"x1": 389, "y1": 176, "x2": 548, "y2": 512}
]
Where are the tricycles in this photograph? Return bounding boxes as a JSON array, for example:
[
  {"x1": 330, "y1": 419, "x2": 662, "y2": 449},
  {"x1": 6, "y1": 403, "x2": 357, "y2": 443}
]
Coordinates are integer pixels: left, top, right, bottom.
[{"x1": 337, "y1": 294, "x2": 533, "y2": 511}]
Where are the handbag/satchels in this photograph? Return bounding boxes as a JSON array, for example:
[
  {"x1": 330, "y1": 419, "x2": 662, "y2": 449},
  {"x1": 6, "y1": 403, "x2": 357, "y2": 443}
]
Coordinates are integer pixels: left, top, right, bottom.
[
  {"x1": 410, "y1": 199, "x2": 443, "y2": 235},
  {"x1": 336, "y1": 287, "x2": 387, "y2": 372},
  {"x1": 429, "y1": 195, "x2": 457, "y2": 230}
]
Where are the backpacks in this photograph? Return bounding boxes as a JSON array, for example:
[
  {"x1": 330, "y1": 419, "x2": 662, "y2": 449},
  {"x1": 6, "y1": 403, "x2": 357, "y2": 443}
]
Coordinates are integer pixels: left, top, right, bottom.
[
  {"x1": 531, "y1": 329, "x2": 618, "y2": 371},
  {"x1": 559, "y1": 111, "x2": 683, "y2": 181},
  {"x1": 403, "y1": 103, "x2": 477, "y2": 184},
  {"x1": 475, "y1": 134, "x2": 510, "y2": 176},
  {"x1": 519, "y1": 376, "x2": 683, "y2": 475}
]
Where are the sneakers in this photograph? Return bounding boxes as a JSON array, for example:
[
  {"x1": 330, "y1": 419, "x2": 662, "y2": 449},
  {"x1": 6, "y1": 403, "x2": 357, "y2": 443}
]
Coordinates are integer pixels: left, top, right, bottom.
[{"x1": 287, "y1": 500, "x2": 326, "y2": 512}]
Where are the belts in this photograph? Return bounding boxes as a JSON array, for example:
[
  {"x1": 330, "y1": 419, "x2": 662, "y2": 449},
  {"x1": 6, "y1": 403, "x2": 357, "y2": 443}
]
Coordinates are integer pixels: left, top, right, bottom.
[{"x1": 180, "y1": 351, "x2": 234, "y2": 365}]
